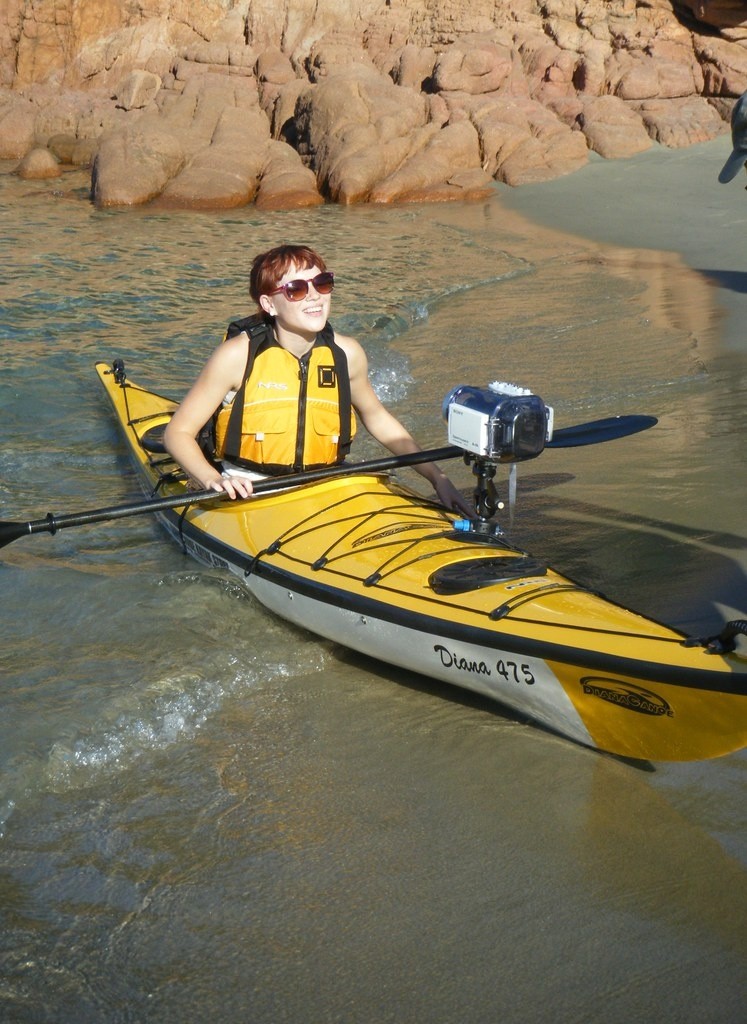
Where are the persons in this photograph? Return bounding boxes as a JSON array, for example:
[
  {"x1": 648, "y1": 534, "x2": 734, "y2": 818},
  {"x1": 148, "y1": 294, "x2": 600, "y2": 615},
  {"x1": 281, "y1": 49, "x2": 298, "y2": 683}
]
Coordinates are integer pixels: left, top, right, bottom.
[
  {"x1": 718, "y1": 89, "x2": 746, "y2": 184},
  {"x1": 162, "y1": 244, "x2": 480, "y2": 523}
]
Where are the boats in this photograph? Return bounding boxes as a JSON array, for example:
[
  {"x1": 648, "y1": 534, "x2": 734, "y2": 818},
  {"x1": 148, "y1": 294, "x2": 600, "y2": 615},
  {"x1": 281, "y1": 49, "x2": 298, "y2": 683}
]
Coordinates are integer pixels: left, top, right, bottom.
[{"x1": 93, "y1": 361, "x2": 747, "y2": 767}]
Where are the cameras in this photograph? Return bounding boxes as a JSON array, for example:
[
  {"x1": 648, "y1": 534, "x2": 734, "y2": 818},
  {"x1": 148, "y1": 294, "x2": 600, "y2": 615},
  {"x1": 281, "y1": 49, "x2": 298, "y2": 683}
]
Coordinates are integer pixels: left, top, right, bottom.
[{"x1": 441, "y1": 385, "x2": 548, "y2": 465}]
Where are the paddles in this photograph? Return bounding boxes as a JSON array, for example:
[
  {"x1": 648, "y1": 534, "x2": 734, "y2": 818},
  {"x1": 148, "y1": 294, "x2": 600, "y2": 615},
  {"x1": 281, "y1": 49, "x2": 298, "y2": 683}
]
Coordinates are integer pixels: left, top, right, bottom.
[{"x1": 2, "y1": 407, "x2": 662, "y2": 549}]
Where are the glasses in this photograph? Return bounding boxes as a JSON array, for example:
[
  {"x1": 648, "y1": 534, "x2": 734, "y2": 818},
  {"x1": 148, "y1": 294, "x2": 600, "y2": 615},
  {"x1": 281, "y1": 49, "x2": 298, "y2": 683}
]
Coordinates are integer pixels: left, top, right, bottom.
[{"x1": 268, "y1": 273, "x2": 336, "y2": 300}]
